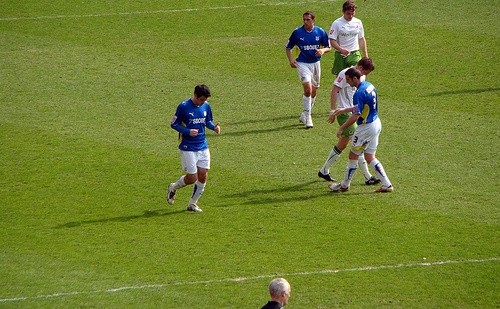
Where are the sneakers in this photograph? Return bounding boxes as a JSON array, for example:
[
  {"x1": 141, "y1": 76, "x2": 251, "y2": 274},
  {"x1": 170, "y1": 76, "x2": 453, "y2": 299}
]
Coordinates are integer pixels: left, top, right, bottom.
[
  {"x1": 318, "y1": 170, "x2": 335, "y2": 181},
  {"x1": 365, "y1": 176, "x2": 381, "y2": 185},
  {"x1": 187, "y1": 203, "x2": 202, "y2": 211},
  {"x1": 167, "y1": 182, "x2": 175, "y2": 205},
  {"x1": 376, "y1": 184, "x2": 393, "y2": 192},
  {"x1": 306, "y1": 117, "x2": 313, "y2": 127},
  {"x1": 328, "y1": 183, "x2": 348, "y2": 191},
  {"x1": 299, "y1": 113, "x2": 305, "y2": 124}
]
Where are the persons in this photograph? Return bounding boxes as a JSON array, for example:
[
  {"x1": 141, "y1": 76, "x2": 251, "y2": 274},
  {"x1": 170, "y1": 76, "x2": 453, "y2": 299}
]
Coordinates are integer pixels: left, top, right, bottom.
[
  {"x1": 286, "y1": 11, "x2": 331, "y2": 128},
  {"x1": 318, "y1": 0, "x2": 393, "y2": 192},
  {"x1": 260, "y1": 278, "x2": 291, "y2": 309},
  {"x1": 167, "y1": 84, "x2": 221, "y2": 211}
]
